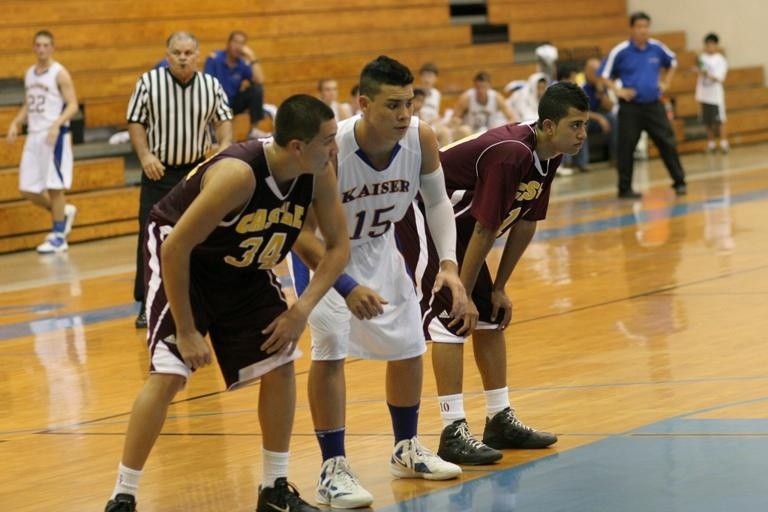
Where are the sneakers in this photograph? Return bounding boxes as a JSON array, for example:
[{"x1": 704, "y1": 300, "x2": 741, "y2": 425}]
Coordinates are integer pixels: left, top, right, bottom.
[
  {"x1": 437, "y1": 419, "x2": 502, "y2": 466},
  {"x1": 388, "y1": 437, "x2": 462, "y2": 481},
  {"x1": 103, "y1": 494, "x2": 137, "y2": 512},
  {"x1": 63, "y1": 204, "x2": 77, "y2": 239},
  {"x1": 482, "y1": 406, "x2": 558, "y2": 451},
  {"x1": 676, "y1": 186, "x2": 687, "y2": 196},
  {"x1": 36, "y1": 232, "x2": 69, "y2": 253},
  {"x1": 315, "y1": 455, "x2": 374, "y2": 509},
  {"x1": 255, "y1": 477, "x2": 320, "y2": 512},
  {"x1": 136, "y1": 303, "x2": 147, "y2": 329},
  {"x1": 617, "y1": 190, "x2": 642, "y2": 199}
]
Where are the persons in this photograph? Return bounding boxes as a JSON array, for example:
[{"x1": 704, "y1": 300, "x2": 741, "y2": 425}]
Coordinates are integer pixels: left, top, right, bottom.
[
  {"x1": 294, "y1": 57, "x2": 465, "y2": 509},
  {"x1": 313, "y1": 42, "x2": 648, "y2": 177},
  {"x1": 106, "y1": 94, "x2": 347, "y2": 511},
  {"x1": 694, "y1": 33, "x2": 730, "y2": 154},
  {"x1": 127, "y1": 29, "x2": 278, "y2": 328},
  {"x1": 5, "y1": 29, "x2": 79, "y2": 254},
  {"x1": 594, "y1": 13, "x2": 686, "y2": 198},
  {"x1": 394, "y1": 81, "x2": 595, "y2": 465}
]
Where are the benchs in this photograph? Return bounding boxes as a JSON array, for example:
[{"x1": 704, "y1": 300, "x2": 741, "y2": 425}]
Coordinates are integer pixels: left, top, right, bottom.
[{"x1": 0, "y1": 0, "x2": 768, "y2": 256}]
